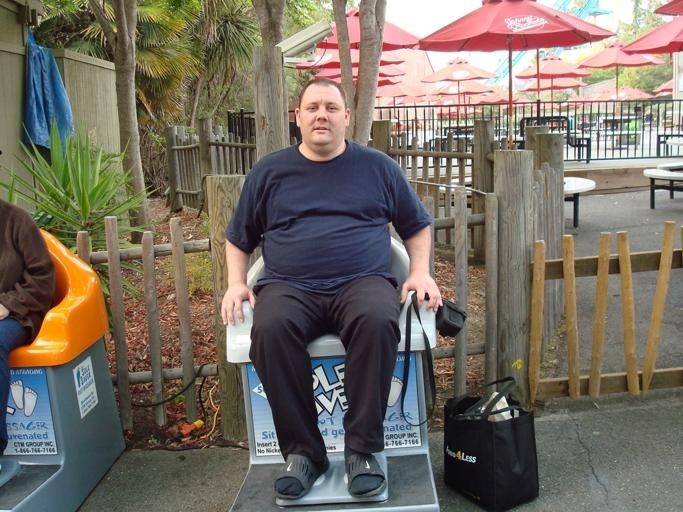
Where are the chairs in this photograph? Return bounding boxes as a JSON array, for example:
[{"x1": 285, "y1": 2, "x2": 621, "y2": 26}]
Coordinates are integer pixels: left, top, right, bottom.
[
  {"x1": 0, "y1": 228, "x2": 127, "y2": 512},
  {"x1": 225, "y1": 235, "x2": 442, "y2": 512}
]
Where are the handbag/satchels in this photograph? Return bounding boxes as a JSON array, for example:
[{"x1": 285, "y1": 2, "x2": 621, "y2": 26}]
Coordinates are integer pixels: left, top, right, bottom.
[{"x1": 443, "y1": 376, "x2": 540, "y2": 512}]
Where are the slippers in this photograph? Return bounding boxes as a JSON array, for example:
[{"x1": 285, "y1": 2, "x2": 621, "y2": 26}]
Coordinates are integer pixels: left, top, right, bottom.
[
  {"x1": 273, "y1": 453, "x2": 330, "y2": 500},
  {"x1": 345, "y1": 453, "x2": 386, "y2": 498}
]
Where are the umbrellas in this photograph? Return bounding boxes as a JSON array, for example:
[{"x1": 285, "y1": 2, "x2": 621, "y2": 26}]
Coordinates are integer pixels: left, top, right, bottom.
[
  {"x1": 620, "y1": 21, "x2": 682, "y2": 57},
  {"x1": 313, "y1": 7, "x2": 419, "y2": 54},
  {"x1": 653, "y1": 0, "x2": 682, "y2": 19},
  {"x1": 420, "y1": 1, "x2": 617, "y2": 151}
]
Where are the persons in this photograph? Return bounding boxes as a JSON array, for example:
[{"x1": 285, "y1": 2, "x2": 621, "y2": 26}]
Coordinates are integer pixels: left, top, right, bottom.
[
  {"x1": 0, "y1": 197, "x2": 59, "y2": 486},
  {"x1": 219, "y1": 75, "x2": 445, "y2": 500}
]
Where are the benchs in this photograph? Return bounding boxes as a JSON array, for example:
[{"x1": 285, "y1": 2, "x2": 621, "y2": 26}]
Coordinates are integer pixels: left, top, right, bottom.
[
  {"x1": 427, "y1": 130, "x2": 682, "y2": 163},
  {"x1": 439, "y1": 175, "x2": 595, "y2": 228},
  {"x1": 642, "y1": 163, "x2": 682, "y2": 209}
]
